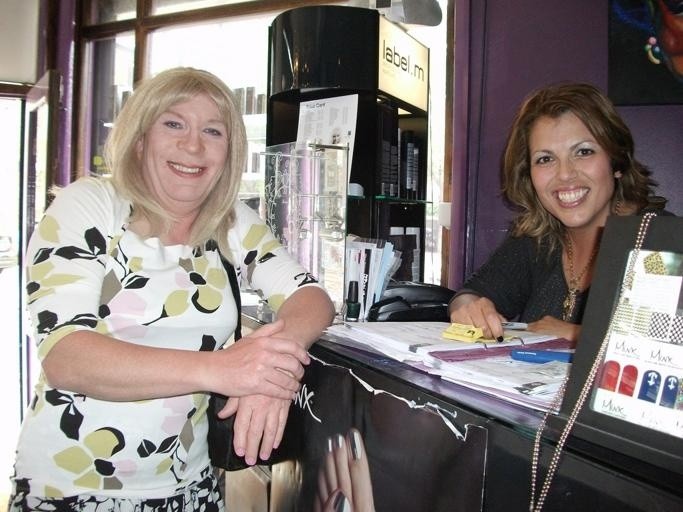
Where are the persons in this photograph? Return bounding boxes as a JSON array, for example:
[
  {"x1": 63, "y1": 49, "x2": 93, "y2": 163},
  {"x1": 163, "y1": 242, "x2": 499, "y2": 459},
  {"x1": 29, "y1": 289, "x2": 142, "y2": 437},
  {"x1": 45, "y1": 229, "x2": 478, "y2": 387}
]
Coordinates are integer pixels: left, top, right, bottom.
[
  {"x1": 312, "y1": 427, "x2": 377, "y2": 511},
  {"x1": 446, "y1": 83, "x2": 676, "y2": 342},
  {"x1": 8, "y1": 66, "x2": 334, "y2": 511}
]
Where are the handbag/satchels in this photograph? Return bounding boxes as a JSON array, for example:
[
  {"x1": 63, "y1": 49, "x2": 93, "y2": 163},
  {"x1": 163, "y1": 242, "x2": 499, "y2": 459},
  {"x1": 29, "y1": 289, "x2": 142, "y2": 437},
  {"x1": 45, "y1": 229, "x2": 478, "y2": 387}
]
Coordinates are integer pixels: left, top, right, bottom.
[{"x1": 205, "y1": 375, "x2": 306, "y2": 471}]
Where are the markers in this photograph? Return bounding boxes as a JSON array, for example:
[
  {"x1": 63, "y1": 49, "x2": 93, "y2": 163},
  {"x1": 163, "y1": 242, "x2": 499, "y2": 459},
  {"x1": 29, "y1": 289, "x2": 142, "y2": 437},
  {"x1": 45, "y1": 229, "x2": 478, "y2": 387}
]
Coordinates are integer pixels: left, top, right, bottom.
[{"x1": 511, "y1": 348, "x2": 575, "y2": 363}]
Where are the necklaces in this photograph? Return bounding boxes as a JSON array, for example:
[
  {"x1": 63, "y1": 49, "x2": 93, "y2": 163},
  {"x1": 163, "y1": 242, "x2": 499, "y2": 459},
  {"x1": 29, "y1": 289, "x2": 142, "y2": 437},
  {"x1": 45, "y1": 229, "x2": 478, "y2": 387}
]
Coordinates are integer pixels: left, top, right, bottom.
[{"x1": 562, "y1": 226, "x2": 601, "y2": 322}]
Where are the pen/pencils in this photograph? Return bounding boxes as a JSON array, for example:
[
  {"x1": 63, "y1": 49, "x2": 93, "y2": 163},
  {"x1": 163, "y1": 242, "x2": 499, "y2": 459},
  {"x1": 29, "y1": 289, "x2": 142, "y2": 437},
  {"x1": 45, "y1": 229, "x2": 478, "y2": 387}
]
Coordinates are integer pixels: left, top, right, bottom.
[{"x1": 501, "y1": 321, "x2": 528, "y2": 329}]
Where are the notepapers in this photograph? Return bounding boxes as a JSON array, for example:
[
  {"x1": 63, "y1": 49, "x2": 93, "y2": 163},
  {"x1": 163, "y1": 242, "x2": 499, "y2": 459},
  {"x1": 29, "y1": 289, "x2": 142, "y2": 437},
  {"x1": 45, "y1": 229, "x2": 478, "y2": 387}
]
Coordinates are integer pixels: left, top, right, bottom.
[{"x1": 443, "y1": 322, "x2": 514, "y2": 345}]
